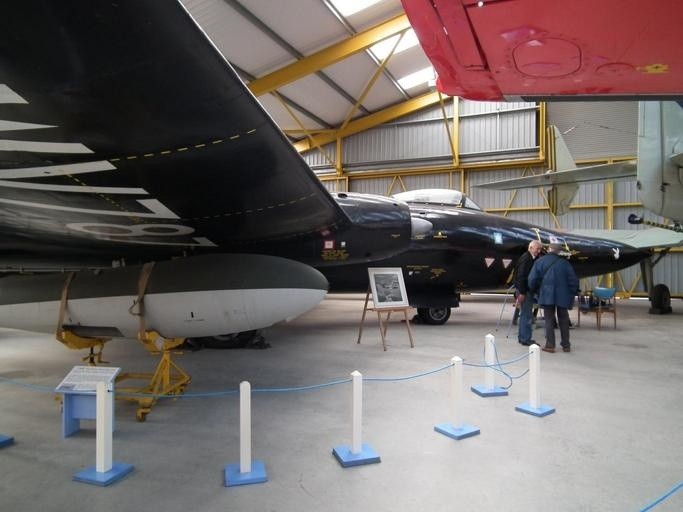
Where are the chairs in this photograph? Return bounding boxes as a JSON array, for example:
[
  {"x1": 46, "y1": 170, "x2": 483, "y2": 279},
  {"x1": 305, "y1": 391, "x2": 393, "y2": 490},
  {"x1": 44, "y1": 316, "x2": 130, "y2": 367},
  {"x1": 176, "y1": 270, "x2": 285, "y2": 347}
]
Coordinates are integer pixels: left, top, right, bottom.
[{"x1": 577, "y1": 286, "x2": 616, "y2": 330}]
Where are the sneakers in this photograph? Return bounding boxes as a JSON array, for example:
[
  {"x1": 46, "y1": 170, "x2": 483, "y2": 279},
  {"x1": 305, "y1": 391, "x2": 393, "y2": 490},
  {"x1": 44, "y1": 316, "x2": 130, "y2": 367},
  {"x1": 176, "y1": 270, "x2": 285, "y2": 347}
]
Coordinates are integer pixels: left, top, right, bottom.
[
  {"x1": 542, "y1": 346, "x2": 554, "y2": 352},
  {"x1": 563, "y1": 346, "x2": 570, "y2": 352},
  {"x1": 519, "y1": 339, "x2": 540, "y2": 346}
]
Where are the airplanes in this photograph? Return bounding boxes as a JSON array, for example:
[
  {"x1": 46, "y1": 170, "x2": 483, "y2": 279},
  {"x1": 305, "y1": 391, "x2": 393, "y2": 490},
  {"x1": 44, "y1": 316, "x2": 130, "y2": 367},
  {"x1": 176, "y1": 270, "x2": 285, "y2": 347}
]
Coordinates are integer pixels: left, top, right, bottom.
[
  {"x1": 0, "y1": 0, "x2": 683, "y2": 350},
  {"x1": 463, "y1": 101, "x2": 681, "y2": 225}
]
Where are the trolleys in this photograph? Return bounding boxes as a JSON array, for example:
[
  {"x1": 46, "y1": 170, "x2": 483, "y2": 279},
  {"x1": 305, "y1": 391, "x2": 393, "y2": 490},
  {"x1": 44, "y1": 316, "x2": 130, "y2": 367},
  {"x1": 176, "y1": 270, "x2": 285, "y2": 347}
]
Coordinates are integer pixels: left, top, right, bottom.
[{"x1": 57, "y1": 326, "x2": 194, "y2": 418}]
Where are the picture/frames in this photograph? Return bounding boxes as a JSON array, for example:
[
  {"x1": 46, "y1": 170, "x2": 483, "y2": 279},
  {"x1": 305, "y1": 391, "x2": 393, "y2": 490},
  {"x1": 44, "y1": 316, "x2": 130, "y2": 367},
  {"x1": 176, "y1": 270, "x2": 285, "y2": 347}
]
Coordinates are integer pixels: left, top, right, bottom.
[{"x1": 367, "y1": 266, "x2": 409, "y2": 309}]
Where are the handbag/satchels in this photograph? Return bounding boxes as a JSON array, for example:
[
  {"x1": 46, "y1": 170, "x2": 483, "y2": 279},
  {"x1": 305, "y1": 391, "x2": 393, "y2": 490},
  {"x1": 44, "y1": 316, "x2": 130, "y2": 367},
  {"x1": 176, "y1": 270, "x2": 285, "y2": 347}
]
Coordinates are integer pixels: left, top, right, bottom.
[{"x1": 533, "y1": 277, "x2": 543, "y2": 295}]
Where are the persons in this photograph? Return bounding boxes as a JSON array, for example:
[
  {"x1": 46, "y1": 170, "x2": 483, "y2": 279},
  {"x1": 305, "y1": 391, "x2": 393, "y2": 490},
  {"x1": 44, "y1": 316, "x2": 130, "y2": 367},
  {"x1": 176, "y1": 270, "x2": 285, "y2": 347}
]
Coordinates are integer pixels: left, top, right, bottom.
[
  {"x1": 513, "y1": 240, "x2": 543, "y2": 346},
  {"x1": 527, "y1": 242, "x2": 579, "y2": 352}
]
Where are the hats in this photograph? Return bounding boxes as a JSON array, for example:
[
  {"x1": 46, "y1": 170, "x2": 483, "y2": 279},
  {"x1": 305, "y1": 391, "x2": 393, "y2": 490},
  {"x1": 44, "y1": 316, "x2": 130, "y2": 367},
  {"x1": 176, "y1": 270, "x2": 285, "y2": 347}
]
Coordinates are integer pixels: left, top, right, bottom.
[{"x1": 549, "y1": 243, "x2": 563, "y2": 250}]
